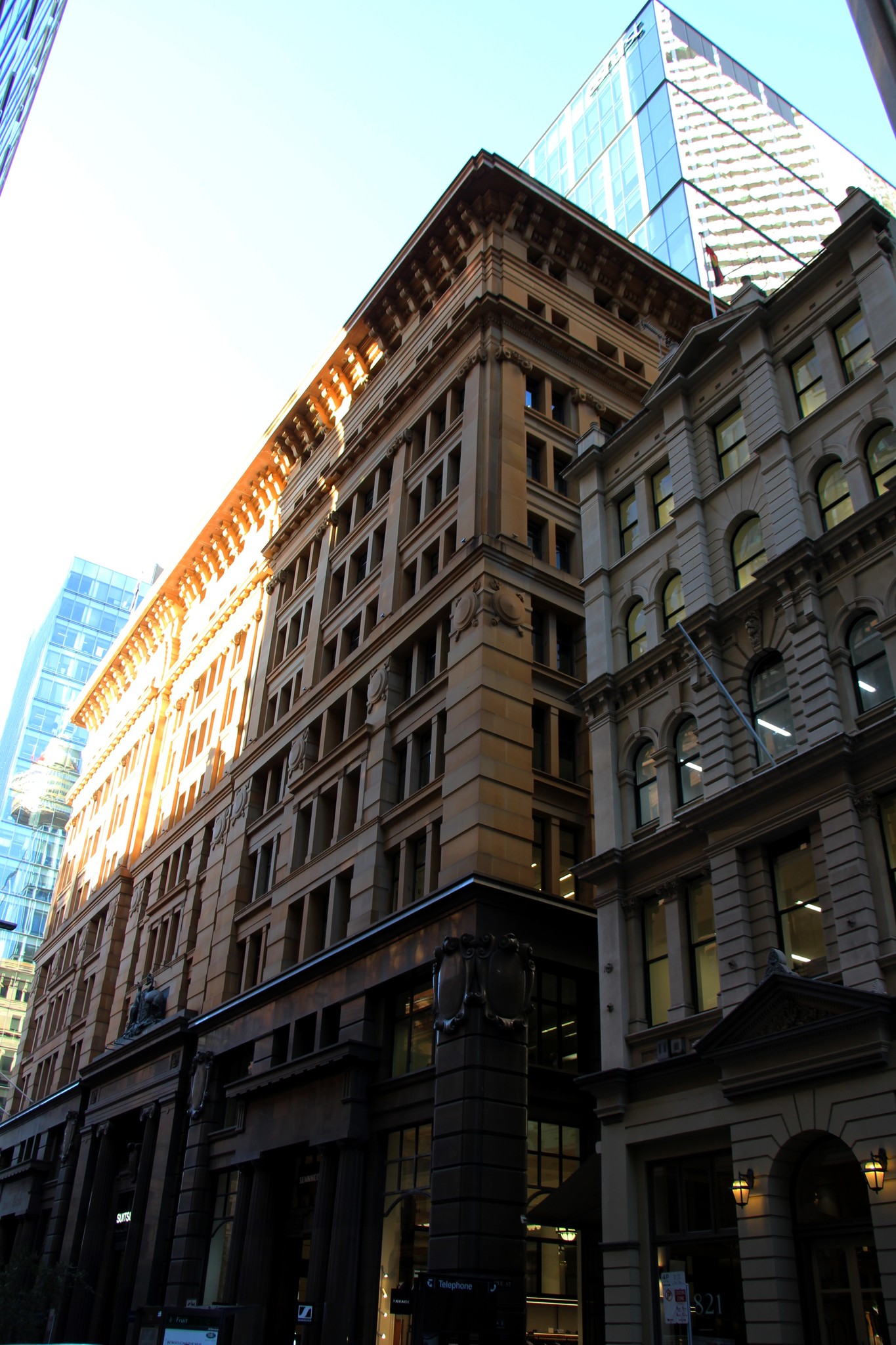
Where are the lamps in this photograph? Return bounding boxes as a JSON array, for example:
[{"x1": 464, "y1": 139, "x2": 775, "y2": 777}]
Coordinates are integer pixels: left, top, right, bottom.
[
  {"x1": 729, "y1": 1167, "x2": 755, "y2": 1209},
  {"x1": 861, "y1": 1147, "x2": 889, "y2": 1195}
]
